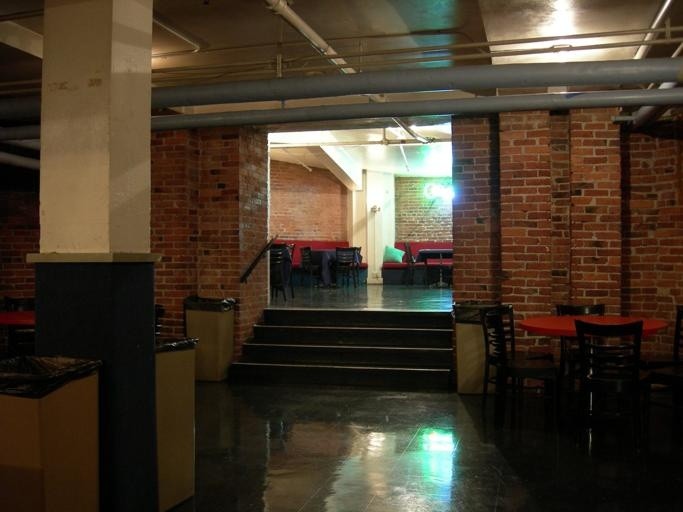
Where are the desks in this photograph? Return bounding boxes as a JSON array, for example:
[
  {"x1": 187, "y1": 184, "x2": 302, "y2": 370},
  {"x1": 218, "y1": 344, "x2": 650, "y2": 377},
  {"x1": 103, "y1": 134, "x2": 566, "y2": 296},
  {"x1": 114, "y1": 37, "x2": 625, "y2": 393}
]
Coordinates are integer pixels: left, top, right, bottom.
[
  {"x1": 0, "y1": 312, "x2": 36, "y2": 341},
  {"x1": 418, "y1": 248, "x2": 454, "y2": 287}
]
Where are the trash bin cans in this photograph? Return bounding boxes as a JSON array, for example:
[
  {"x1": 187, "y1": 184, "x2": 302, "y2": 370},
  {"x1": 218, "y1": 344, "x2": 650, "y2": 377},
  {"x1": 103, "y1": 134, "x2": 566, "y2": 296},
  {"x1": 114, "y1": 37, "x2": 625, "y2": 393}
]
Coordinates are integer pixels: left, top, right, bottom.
[
  {"x1": 183, "y1": 295, "x2": 235, "y2": 381},
  {"x1": 452, "y1": 301, "x2": 502, "y2": 394},
  {"x1": 0, "y1": 355, "x2": 102, "y2": 512},
  {"x1": 154, "y1": 338, "x2": 198, "y2": 512}
]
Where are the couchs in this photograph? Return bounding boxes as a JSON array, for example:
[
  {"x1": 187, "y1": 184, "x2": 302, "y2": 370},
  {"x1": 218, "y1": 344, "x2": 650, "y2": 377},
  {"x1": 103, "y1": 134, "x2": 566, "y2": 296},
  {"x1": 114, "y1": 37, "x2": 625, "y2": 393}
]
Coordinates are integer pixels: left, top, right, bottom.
[
  {"x1": 381, "y1": 242, "x2": 453, "y2": 288},
  {"x1": 273, "y1": 240, "x2": 366, "y2": 287}
]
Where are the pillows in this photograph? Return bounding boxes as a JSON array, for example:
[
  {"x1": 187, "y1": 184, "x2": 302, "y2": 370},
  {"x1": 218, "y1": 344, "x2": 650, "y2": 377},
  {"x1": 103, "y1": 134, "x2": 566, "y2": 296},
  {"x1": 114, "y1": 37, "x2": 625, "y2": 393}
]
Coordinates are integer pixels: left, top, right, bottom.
[{"x1": 384, "y1": 244, "x2": 405, "y2": 263}]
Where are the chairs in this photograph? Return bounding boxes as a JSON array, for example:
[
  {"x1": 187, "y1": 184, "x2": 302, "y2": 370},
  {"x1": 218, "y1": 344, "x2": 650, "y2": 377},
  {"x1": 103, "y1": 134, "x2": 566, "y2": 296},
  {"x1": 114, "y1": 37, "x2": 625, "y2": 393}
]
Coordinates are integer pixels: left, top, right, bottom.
[
  {"x1": 479, "y1": 302, "x2": 683, "y2": 458},
  {"x1": 403, "y1": 243, "x2": 429, "y2": 286},
  {"x1": 6, "y1": 296, "x2": 37, "y2": 358},
  {"x1": 270, "y1": 244, "x2": 361, "y2": 293}
]
